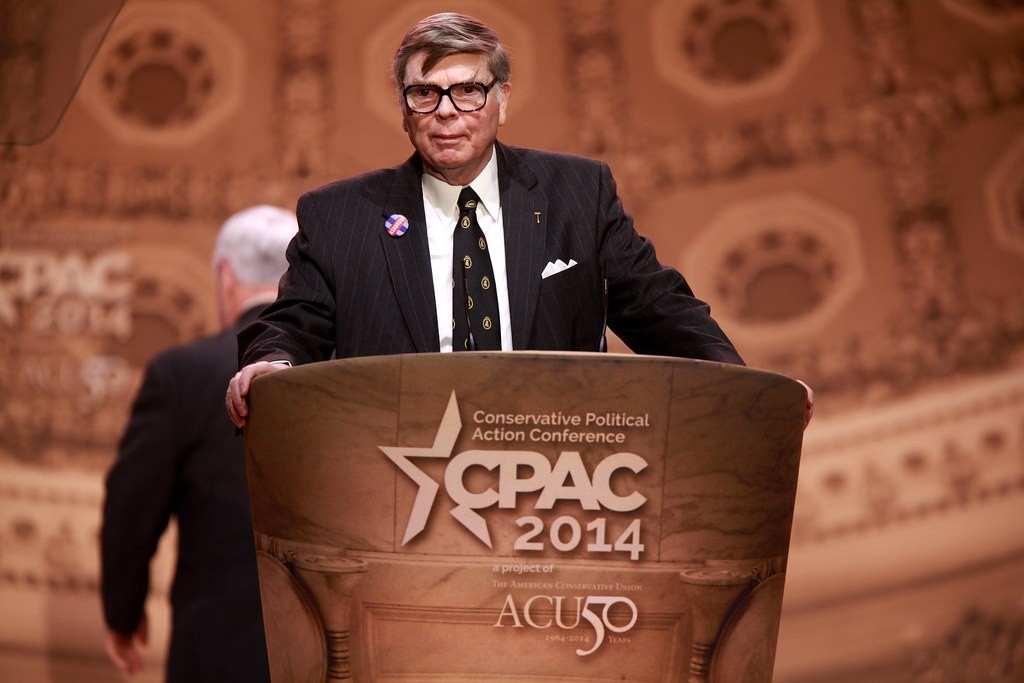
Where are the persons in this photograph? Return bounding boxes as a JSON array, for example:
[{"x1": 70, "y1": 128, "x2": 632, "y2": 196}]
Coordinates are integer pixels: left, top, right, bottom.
[
  {"x1": 225, "y1": 12, "x2": 815, "y2": 430},
  {"x1": 100, "y1": 203, "x2": 302, "y2": 683}
]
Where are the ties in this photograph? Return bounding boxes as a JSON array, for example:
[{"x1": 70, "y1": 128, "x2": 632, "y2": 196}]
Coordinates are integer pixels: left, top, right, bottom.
[{"x1": 451, "y1": 186, "x2": 502, "y2": 352}]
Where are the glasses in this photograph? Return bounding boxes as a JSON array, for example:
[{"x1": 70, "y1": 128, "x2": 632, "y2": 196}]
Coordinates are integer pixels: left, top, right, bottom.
[{"x1": 402, "y1": 78, "x2": 497, "y2": 115}]
